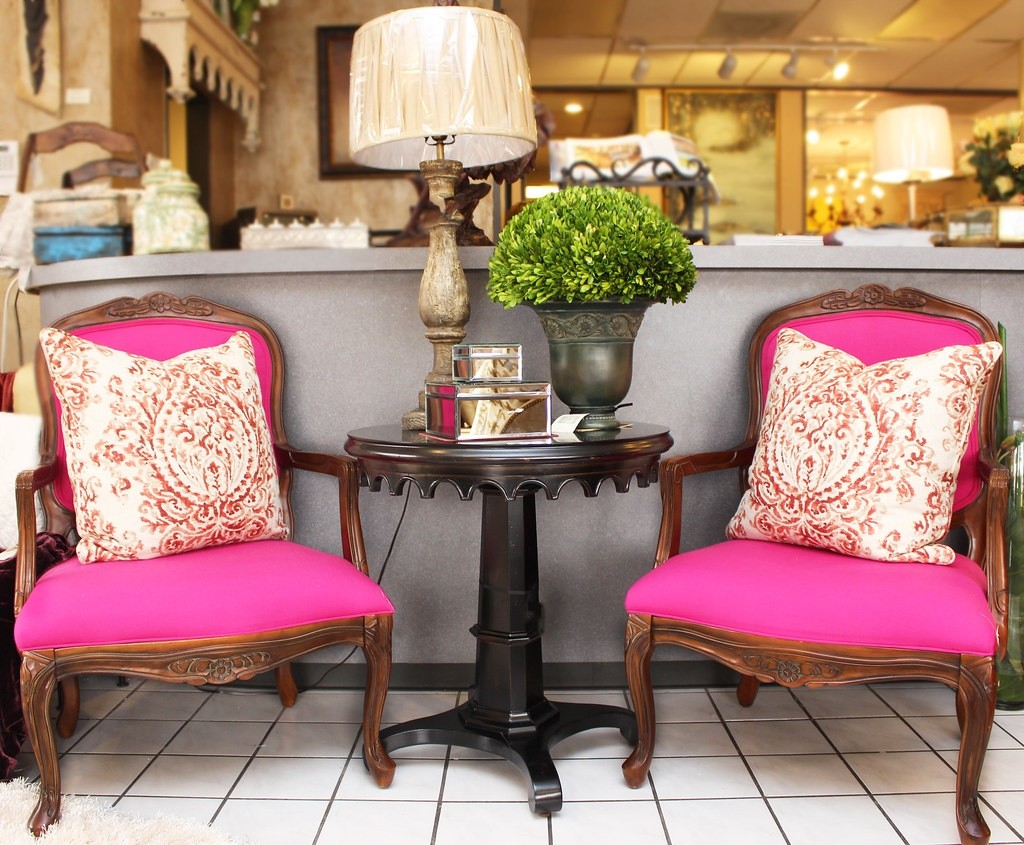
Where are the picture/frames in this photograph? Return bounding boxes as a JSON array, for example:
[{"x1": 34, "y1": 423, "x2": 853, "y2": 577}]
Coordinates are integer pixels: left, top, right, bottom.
[{"x1": 662, "y1": 88, "x2": 781, "y2": 236}]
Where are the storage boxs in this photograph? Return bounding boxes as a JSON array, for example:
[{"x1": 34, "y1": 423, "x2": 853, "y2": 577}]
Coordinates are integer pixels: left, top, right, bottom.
[
  {"x1": 425, "y1": 378, "x2": 552, "y2": 441},
  {"x1": 33, "y1": 227, "x2": 131, "y2": 265},
  {"x1": 452, "y1": 342, "x2": 523, "y2": 383},
  {"x1": 31, "y1": 192, "x2": 123, "y2": 227}
]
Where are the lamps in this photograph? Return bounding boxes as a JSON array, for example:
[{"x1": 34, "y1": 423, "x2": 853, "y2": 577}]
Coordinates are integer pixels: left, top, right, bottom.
[
  {"x1": 349, "y1": 4, "x2": 536, "y2": 430},
  {"x1": 870, "y1": 106, "x2": 953, "y2": 221}
]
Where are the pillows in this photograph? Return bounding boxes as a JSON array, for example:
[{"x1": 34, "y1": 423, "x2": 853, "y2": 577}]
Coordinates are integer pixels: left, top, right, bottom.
[
  {"x1": 726, "y1": 328, "x2": 1001, "y2": 566},
  {"x1": 40, "y1": 328, "x2": 289, "y2": 567}
]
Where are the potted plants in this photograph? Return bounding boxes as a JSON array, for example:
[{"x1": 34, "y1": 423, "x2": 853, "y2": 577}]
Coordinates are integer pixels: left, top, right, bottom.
[{"x1": 485, "y1": 185, "x2": 697, "y2": 432}]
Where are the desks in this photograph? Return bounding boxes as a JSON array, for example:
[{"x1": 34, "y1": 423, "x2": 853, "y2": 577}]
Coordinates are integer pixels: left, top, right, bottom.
[{"x1": 344, "y1": 421, "x2": 674, "y2": 814}]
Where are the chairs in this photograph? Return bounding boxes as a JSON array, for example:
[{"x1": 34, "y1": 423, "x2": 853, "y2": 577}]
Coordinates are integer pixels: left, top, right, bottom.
[
  {"x1": 619, "y1": 284, "x2": 1024, "y2": 845},
  {"x1": 15, "y1": 290, "x2": 397, "y2": 839}
]
